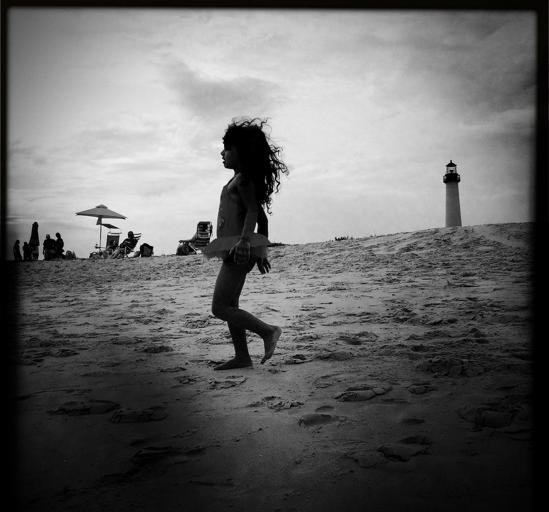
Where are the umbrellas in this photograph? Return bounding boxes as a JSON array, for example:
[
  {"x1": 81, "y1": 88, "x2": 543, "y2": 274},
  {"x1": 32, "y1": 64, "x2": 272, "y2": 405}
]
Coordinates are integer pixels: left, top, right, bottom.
[
  {"x1": 29, "y1": 222, "x2": 40, "y2": 246},
  {"x1": 75, "y1": 204, "x2": 128, "y2": 252}
]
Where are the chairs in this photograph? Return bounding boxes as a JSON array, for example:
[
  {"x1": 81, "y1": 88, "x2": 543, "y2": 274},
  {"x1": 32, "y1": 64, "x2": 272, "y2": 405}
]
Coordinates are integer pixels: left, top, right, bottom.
[
  {"x1": 90, "y1": 231, "x2": 122, "y2": 259},
  {"x1": 179, "y1": 221, "x2": 212, "y2": 254},
  {"x1": 115, "y1": 233, "x2": 141, "y2": 259}
]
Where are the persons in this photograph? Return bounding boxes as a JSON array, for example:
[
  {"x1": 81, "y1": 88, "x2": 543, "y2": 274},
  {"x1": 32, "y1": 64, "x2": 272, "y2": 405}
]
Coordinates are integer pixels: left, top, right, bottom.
[
  {"x1": 22, "y1": 241, "x2": 30, "y2": 259},
  {"x1": 202, "y1": 119, "x2": 291, "y2": 369},
  {"x1": 13, "y1": 240, "x2": 22, "y2": 261},
  {"x1": 43, "y1": 233, "x2": 56, "y2": 261},
  {"x1": 176, "y1": 222, "x2": 210, "y2": 255},
  {"x1": 33, "y1": 245, "x2": 39, "y2": 260},
  {"x1": 108, "y1": 231, "x2": 137, "y2": 258},
  {"x1": 54, "y1": 232, "x2": 66, "y2": 259}
]
